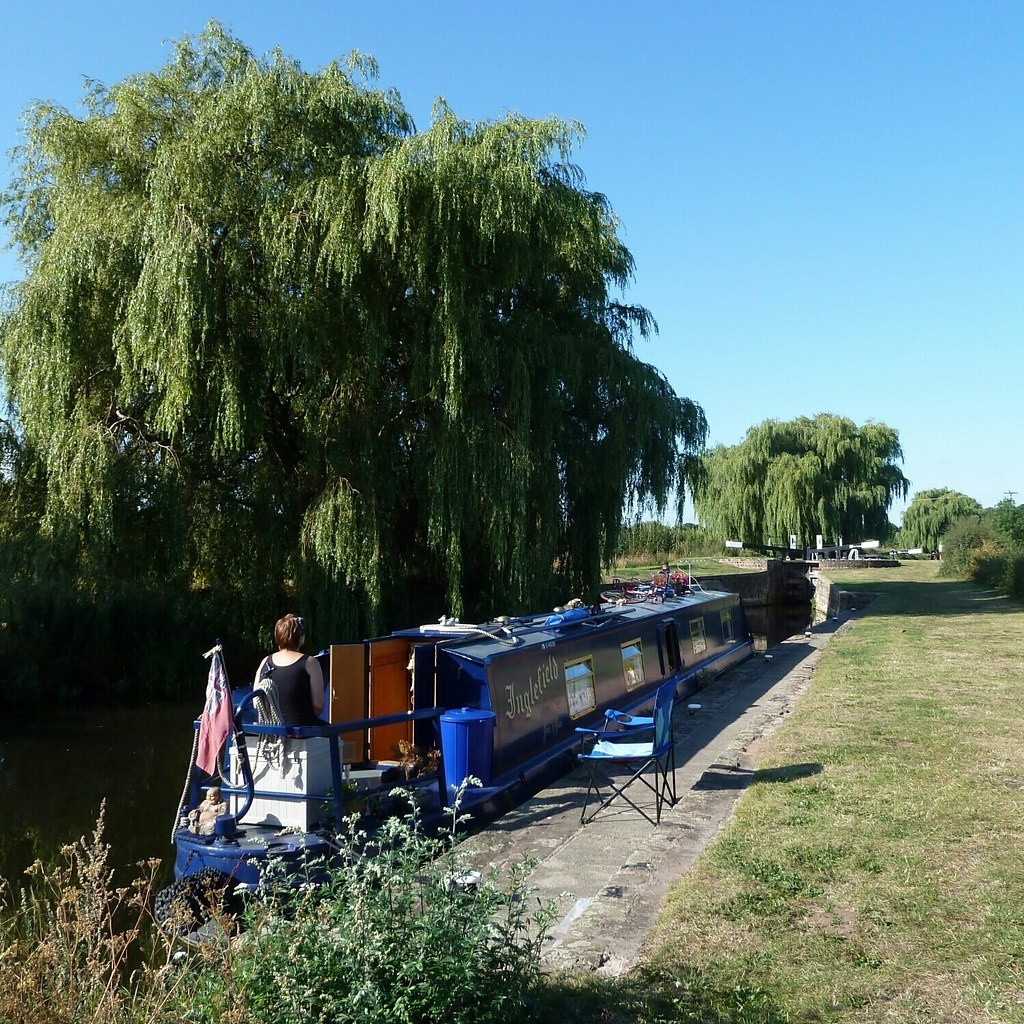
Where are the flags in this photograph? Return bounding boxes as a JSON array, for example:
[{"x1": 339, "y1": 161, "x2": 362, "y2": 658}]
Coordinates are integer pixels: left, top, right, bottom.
[{"x1": 195, "y1": 650, "x2": 233, "y2": 778}]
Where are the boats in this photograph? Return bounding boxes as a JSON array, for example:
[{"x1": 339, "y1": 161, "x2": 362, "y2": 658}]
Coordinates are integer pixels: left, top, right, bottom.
[{"x1": 157, "y1": 562, "x2": 755, "y2": 948}]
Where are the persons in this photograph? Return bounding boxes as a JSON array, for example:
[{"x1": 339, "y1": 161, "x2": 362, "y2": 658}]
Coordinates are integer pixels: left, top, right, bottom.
[
  {"x1": 938, "y1": 540, "x2": 944, "y2": 560},
  {"x1": 253, "y1": 615, "x2": 341, "y2": 738}
]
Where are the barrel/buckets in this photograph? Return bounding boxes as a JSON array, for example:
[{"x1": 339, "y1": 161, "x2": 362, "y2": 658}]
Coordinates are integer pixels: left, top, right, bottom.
[{"x1": 440, "y1": 707, "x2": 496, "y2": 808}]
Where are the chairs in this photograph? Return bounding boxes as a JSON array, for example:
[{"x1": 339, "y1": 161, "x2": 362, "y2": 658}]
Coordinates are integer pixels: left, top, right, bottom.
[{"x1": 574, "y1": 675, "x2": 680, "y2": 826}]
